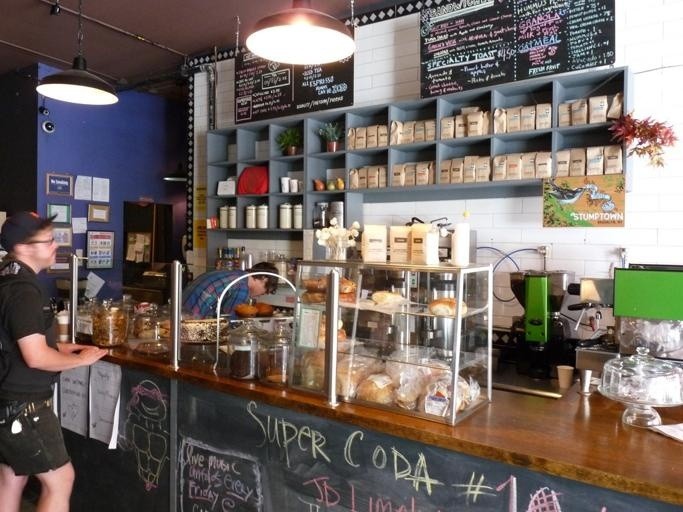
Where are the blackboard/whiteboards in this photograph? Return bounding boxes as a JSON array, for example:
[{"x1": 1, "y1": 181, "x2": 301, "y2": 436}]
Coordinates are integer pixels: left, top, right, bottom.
[
  {"x1": 235, "y1": 24, "x2": 354, "y2": 124},
  {"x1": 22, "y1": 362, "x2": 683, "y2": 512},
  {"x1": 420, "y1": 0, "x2": 615, "y2": 99}
]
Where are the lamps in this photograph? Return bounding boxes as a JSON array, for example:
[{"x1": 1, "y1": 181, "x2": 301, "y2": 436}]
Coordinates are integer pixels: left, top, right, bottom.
[
  {"x1": 244, "y1": 0, "x2": 355, "y2": 67},
  {"x1": 35, "y1": 0, "x2": 119, "y2": 106},
  {"x1": 162, "y1": 161, "x2": 187, "y2": 183}
]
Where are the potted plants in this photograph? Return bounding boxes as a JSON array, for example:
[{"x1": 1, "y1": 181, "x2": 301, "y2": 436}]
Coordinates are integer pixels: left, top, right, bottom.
[
  {"x1": 315, "y1": 121, "x2": 344, "y2": 152},
  {"x1": 275, "y1": 128, "x2": 303, "y2": 155}
]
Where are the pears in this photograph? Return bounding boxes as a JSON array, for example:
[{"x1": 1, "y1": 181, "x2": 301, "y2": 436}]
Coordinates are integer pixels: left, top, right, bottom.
[{"x1": 313, "y1": 177, "x2": 344, "y2": 191}]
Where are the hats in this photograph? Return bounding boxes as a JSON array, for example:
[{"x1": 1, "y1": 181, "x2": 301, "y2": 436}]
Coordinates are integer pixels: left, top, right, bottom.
[{"x1": 0, "y1": 210, "x2": 58, "y2": 250}]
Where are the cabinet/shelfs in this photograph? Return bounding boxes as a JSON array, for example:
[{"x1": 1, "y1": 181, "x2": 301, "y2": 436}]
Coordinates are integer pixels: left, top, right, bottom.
[
  {"x1": 288, "y1": 259, "x2": 494, "y2": 428},
  {"x1": 205, "y1": 66, "x2": 628, "y2": 274}
]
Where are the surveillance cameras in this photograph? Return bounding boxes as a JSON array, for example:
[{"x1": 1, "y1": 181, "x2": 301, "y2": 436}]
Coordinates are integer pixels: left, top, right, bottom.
[
  {"x1": 42, "y1": 121, "x2": 56, "y2": 133},
  {"x1": 39, "y1": 105, "x2": 51, "y2": 118}
]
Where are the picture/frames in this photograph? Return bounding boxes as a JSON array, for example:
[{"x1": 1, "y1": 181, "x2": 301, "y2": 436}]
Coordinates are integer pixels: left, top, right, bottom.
[
  {"x1": 87, "y1": 205, "x2": 110, "y2": 223},
  {"x1": 46, "y1": 252, "x2": 72, "y2": 275},
  {"x1": 46, "y1": 172, "x2": 74, "y2": 197},
  {"x1": 86, "y1": 229, "x2": 115, "y2": 270},
  {"x1": 53, "y1": 226, "x2": 73, "y2": 247},
  {"x1": 46, "y1": 203, "x2": 72, "y2": 224}
]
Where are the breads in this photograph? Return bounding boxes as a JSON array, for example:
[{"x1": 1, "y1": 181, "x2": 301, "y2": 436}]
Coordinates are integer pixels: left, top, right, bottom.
[{"x1": 300, "y1": 275, "x2": 474, "y2": 417}]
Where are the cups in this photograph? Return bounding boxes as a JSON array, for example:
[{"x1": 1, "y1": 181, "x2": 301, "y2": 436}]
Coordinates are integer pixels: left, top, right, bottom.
[
  {"x1": 579, "y1": 368, "x2": 592, "y2": 392},
  {"x1": 280, "y1": 177, "x2": 299, "y2": 193},
  {"x1": 219, "y1": 202, "x2": 303, "y2": 229},
  {"x1": 556, "y1": 365, "x2": 575, "y2": 389},
  {"x1": 56, "y1": 309, "x2": 72, "y2": 343}
]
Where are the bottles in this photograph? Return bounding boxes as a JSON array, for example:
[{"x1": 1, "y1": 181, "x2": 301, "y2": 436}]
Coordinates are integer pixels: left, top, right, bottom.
[
  {"x1": 227, "y1": 317, "x2": 268, "y2": 381},
  {"x1": 257, "y1": 319, "x2": 292, "y2": 390},
  {"x1": 313, "y1": 202, "x2": 330, "y2": 229},
  {"x1": 90, "y1": 297, "x2": 173, "y2": 348}
]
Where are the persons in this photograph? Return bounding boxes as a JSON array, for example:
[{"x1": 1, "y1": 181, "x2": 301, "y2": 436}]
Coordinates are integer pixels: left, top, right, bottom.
[
  {"x1": 0, "y1": 211, "x2": 110, "y2": 511},
  {"x1": 182, "y1": 262, "x2": 278, "y2": 320}
]
